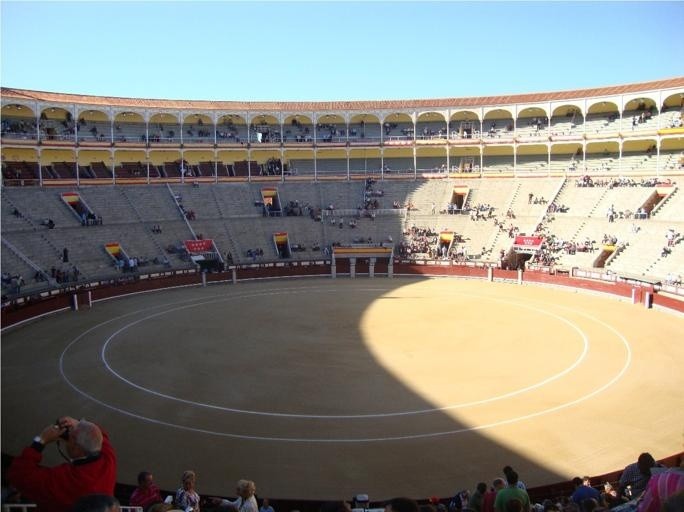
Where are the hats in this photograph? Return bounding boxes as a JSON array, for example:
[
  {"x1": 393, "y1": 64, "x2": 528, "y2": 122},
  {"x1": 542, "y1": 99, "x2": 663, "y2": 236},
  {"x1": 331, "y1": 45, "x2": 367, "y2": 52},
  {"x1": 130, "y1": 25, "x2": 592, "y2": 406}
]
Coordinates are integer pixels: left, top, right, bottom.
[{"x1": 639, "y1": 453, "x2": 656, "y2": 473}]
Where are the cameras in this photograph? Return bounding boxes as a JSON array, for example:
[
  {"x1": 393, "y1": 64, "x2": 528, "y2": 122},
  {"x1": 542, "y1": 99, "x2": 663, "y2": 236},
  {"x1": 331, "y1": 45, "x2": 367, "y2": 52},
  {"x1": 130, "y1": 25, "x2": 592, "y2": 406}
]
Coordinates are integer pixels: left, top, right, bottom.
[{"x1": 54, "y1": 417, "x2": 70, "y2": 443}]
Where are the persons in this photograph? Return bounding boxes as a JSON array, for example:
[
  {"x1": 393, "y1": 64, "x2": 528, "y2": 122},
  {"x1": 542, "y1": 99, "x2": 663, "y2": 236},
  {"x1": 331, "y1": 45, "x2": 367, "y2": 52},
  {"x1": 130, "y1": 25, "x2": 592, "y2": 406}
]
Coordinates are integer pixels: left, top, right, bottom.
[
  {"x1": 341, "y1": 453, "x2": 683, "y2": 512},
  {"x1": 2, "y1": 108, "x2": 683, "y2": 301},
  {"x1": 0, "y1": 414, "x2": 304, "y2": 512}
]
